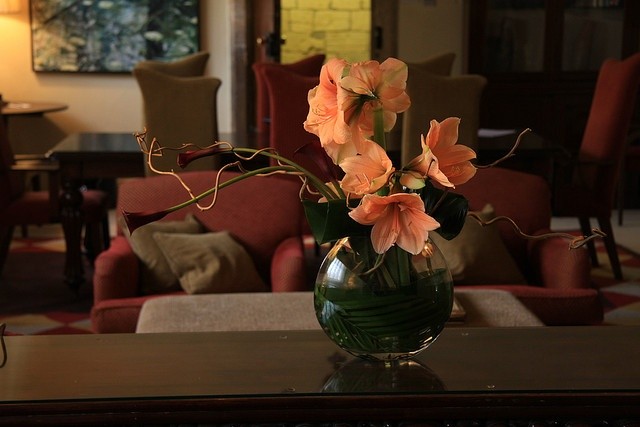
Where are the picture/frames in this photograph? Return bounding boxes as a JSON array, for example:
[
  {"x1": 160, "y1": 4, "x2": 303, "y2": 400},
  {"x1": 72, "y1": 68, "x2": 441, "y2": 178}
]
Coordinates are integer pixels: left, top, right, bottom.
[{"x1": 28, "y1": 0, "x2": 201, "y2": 74}]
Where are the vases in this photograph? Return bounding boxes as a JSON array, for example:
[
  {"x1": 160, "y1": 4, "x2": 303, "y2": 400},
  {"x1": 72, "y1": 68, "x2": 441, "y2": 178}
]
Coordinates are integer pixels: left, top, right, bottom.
[{"x1": 314, "y1": 237, "x2": 455, "y2": 361}]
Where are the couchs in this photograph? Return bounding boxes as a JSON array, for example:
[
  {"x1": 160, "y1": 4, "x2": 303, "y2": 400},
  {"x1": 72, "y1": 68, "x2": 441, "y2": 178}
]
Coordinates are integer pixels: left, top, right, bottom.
[
  {"x1": 429, "y1": 168, "x2": 602, "y2": 327},
  {"x1": 91, "y1": 169, "x2": 305, "y2": 332}
]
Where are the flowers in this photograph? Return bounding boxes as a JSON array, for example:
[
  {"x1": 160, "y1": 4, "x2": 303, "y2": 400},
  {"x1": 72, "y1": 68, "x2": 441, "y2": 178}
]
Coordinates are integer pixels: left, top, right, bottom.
[{"x1": 123, "y1": 57, "x2": 608, "y2": 256}]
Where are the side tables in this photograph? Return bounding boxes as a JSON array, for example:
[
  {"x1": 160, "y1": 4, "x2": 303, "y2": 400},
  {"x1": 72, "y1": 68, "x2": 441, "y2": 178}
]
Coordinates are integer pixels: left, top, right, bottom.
[{"x1": 47, "y1": 131, "x2": 142, "y2": 278}]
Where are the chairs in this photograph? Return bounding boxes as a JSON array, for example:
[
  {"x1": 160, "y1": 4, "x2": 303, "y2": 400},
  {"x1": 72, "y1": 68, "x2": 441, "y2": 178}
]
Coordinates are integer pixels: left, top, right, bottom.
[
  {"x1": 256, "y1": 52, "x2": 326, "y2": 164},
  {"x1": 1, "y1": 116, "x2": 108, "y2": 269},
  {"x1": 266, "y1": 65, "x2": 322, "y2": 177},
  {"x1": 400, "y1": 72, "x2": 485, "y2": 165},
  {"x1": 511, "y1": 56, "x2": 640, "y2": 281},
  {"x1": 134, "y1": 67, "x2": 220, "y2": 171},
  {"x1": 140, "y1": 50, "x2": 209, "y2": 76}
]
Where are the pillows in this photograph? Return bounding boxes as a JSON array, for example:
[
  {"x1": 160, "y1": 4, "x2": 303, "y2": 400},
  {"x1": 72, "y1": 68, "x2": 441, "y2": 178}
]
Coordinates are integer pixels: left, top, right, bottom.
[
  {"x1": 415, "y1": 205, "x2": 525, "y2": 283},
  {"x1": 121, "y1": 214, "x2": 202, "y2": 290},
  {"x1": 152, "y1": 231, "x2": 268, "y2": 295}
]
